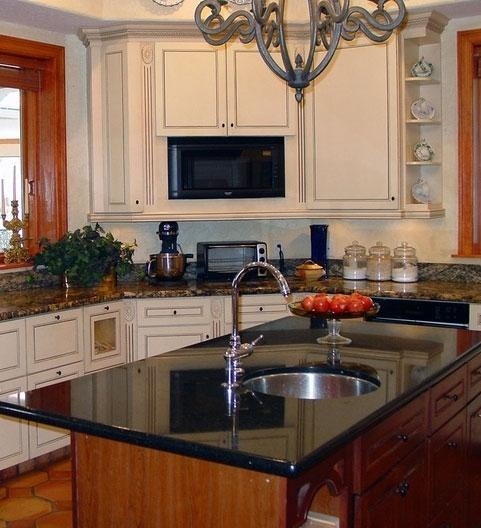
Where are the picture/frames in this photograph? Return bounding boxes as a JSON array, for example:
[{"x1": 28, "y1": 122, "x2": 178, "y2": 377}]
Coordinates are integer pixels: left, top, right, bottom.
[{"x1": 457, "y1": 31, "x2": 481, "y2": 258}]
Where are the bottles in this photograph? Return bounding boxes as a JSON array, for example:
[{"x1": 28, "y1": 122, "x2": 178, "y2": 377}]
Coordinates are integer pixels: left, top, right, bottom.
[
  {"x1": 342, "y1": 239, "x2": 367, "y2": 280},
  {"x1": 393, "y1": 241, "x2": 418, "y2": 284},
  {"x1": 366, "y1": 240, "x2": 393, "y2": 281}
]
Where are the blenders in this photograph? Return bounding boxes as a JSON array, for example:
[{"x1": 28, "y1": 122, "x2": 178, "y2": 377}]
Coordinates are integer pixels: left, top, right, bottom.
[{"x1": 145, "y1": 221, "x2": 195, "y2": 288}]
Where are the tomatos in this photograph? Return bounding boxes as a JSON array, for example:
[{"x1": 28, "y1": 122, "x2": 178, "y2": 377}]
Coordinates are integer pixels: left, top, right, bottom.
[{"x1": 301, "y1": 292, "x2": 373, "y2": 314}]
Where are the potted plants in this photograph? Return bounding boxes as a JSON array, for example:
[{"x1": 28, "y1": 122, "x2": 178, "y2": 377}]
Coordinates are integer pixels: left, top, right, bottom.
[{"x1": 31, "y1": 223, "x2": 137, "y2": 289}]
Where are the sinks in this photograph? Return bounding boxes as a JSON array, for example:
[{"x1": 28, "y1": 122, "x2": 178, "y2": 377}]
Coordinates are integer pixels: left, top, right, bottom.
[{"x1": 244, "y1": 372, "x2": 382, "y2": 400}]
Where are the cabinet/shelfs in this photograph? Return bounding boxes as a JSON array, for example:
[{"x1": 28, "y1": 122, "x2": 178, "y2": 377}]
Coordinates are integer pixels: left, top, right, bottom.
[
  {"x1": 353, "y1": 390, "x2": 431, "y2": 528},
  {"x1": 404, "y1": 77, "x2": 442, "y2": 210},
  {"x1": 238, "y1": 295, "x2": 315, "y2": 332},
  {"x1": 431, "y1": 360, "x2": 467, "y2": 518},
  {"x1": 29, "y1": 306, "x2": 84, "y2": 473},
  {"x1": 88, "y1": 38, "x2": 146, "y2": 215},
  {"x1": 137, "y1": 296, "x2": 227, "y2": 362},
  {"x1": 84, "y1": 300, "x2": 127, "y2": 374},
  {"x1": 468, "y1": 349, "x2": 479, "y2": 526},
  {"x1": 0, "y1": 314, "x2": 28, "y2": 477},
  {"x1": 312, "y1": 44, "x2": 391, "y2": 202},
  {"x1": 165, "y1": 49, "x2": 288, "y2": 128}
]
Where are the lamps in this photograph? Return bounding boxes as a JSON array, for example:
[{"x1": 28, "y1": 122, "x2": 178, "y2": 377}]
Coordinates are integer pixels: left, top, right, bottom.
[{"x1": 194, "y1": 1, "x2": 408, "y2": 103}]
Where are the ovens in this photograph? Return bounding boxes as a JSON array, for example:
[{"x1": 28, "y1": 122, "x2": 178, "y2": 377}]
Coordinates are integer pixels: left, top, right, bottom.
[{"x1": 167, "y1": 136, "x2": 287, "y2": 200}]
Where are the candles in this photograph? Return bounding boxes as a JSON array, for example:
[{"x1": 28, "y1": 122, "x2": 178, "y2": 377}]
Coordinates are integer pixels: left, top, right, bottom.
[
  {"x1": 12, "y1": 165, "x2": 17, "y2": 202},
  {"x1": 24, "y1": 178, "x2": 29, "y2": 215},
  {"x1": 1, "y1": 179, "x2": 5, "y2": 214}
]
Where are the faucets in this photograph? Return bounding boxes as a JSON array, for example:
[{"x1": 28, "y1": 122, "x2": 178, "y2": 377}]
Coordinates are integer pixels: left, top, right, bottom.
[
  {"x1": 220, "y1": 361, "x2": 264, "y2": 450},
  {"x1": 223, "y1": 261, "x2": 291, "y2": 361}
]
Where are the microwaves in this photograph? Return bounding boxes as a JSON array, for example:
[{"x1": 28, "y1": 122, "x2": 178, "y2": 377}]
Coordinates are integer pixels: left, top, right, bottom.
[{"x1": 197, "y1": 240, "x2": 269, "y2": 280}]
